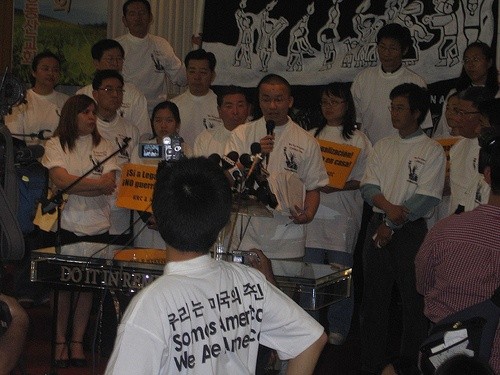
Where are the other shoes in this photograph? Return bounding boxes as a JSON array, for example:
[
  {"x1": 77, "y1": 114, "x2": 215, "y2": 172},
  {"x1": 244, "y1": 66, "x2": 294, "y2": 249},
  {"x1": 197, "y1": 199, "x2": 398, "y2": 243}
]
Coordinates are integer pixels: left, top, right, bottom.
[{"x1": 329, "y1": 333, "x2": 346, "y2": 345}]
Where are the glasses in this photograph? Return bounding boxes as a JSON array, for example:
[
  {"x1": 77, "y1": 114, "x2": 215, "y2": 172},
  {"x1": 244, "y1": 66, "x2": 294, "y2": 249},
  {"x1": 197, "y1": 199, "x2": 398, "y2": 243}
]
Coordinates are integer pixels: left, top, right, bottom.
[
  {"x1": 376, "y1": 44, "x2": 402, "y2": 54},
  {"x1": 388, "y1": 106, "x2": 411, "y2": 112},
  {"x1": 98, "y1": 88, "x2": 125, "y2": 94},
  {"x1": 457, "y1": 109, "x2": 481, "y2": 115},
  {"x1": 320, "y1": 100, "x2": 345, "y2": 106},
  {"x1": 100, "y1": 55, "x2": 124, "y2": 63}
]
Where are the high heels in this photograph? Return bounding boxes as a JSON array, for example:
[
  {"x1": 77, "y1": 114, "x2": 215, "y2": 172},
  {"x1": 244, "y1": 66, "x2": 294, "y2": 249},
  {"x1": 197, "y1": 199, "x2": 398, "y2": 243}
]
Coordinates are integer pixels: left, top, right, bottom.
[
  {"x1": 53, "y1": 340, "x2": 70, "y2": 369},
  {"x1": 67, "y1": 338, "x2": 88, "y2": 367}
]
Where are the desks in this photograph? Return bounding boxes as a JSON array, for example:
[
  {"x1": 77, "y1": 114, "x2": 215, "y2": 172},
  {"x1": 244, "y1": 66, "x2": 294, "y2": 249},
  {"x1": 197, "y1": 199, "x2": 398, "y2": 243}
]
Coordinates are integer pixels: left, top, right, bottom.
[{"x1": 28, "y1": 242, "x2": 355, "y2": 375}]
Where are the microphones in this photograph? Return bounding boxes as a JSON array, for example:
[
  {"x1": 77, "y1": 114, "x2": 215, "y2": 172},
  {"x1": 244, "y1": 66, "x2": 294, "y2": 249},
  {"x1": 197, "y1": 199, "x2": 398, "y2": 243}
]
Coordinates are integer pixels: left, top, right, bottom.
[
  {"x1": 209, "y1": 140, "x2": 268, "y2": 194},
  {"x1": 264, "y1": 119, "x2": 275, "y2": 164}
]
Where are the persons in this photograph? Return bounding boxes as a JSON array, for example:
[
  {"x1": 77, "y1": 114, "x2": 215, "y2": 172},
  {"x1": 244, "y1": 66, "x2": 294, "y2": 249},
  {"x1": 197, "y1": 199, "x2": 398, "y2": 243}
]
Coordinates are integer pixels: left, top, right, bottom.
[
  {"x1": 112, "y1": 0, "x2": 205, "y2": 118},
  {"x1": 0, "y1": 25, "x2": 500, "y2": 375}
]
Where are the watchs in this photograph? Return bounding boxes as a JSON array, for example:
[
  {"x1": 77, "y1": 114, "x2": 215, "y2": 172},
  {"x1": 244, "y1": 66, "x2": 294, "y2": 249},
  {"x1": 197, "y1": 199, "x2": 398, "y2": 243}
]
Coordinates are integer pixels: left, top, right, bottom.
[{"x1": 384, "y1": 220, "x2": 390, "y2": 227}]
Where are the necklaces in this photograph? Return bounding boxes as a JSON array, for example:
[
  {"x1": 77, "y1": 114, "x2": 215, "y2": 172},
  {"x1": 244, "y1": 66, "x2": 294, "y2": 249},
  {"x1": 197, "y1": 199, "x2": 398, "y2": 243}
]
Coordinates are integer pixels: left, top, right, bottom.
[{"x1": 97, "y1": 109, "x2": 111, "y2": 122}]
